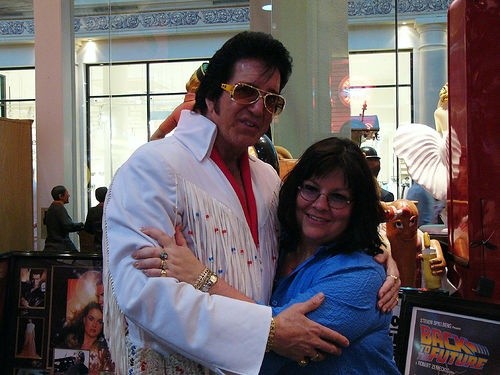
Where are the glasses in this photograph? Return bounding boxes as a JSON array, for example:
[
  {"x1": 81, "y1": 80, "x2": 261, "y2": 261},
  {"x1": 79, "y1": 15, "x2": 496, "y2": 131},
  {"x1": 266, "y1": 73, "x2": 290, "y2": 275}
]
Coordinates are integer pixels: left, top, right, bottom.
[
  {"x1": 297, "y1": 185, "x2": 353, "y2": 210},
  {"x1": 220, "y1": 83, "x2": 285, "y2": 115}
]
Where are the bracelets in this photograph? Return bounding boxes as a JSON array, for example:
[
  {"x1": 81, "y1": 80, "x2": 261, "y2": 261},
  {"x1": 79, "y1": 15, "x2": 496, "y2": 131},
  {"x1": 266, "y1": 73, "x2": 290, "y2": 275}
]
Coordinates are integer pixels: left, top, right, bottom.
[
  {"x1": 266, "y1": 317, "x2": 276, "y2": 353},
  {"x1": 193, "y1": 266, "x2": 218, "y2": 291}
]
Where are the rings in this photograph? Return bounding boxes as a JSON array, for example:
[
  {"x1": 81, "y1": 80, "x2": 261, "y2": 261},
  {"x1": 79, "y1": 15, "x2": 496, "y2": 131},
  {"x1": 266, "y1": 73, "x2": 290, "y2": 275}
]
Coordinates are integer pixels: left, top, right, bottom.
[
  {"x1": 386, "y1": 274, "x2": 399, "y2": 281},
  {"x1": 298, "y1": 361, "x2": 307, "y2": 367},
  {"x1": 159, "y1": 247, "x2": 168, "y2": 277},
  {"x1": 310, "y1": 354, "x2": 319, "y2": 361}
]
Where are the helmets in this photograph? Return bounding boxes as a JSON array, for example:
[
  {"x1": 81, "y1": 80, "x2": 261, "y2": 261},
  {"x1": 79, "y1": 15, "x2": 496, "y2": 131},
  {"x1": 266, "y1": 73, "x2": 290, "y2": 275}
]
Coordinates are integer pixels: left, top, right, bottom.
[{"x1": 359, "y1": 147, "x2": 381, "y2": 159}]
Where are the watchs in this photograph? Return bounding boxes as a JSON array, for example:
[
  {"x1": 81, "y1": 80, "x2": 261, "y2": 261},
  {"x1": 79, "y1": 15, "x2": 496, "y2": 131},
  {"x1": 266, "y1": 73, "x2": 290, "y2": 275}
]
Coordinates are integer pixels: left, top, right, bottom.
[{"x1": 201, "y1": 271, "x2": 219, "y2": 292}]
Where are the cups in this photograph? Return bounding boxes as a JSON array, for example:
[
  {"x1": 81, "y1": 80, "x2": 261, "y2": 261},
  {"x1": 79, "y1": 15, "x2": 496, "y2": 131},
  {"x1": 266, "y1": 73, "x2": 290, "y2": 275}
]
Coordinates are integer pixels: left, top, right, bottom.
[{"x1": 422, "y1": 250, "x2": 442, "y2": 289}]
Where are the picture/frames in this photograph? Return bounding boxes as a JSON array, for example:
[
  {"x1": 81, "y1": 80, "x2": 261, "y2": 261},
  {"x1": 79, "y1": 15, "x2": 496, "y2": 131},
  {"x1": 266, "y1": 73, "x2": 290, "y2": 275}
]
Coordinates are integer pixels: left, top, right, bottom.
[
  {"x1": 393, "y1": 291, "x2": 500, "y2": 375},
  {"x1": 0, "y1": 254, "x2": 115, "y2": 375}
]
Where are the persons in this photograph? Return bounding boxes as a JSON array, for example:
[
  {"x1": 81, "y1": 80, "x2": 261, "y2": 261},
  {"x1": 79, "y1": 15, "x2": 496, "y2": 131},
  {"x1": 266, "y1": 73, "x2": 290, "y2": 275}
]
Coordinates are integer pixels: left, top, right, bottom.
[
  {"x1": 42, "y1": 185, "x2": 85, "y2": 252},
  {"x1": 14, "y1": 267, "x2": 114, "y2": 374},
  {"x1": 99, "y1": 30, "x2": 402, "y2": 375},
  {"x1": 85, "y1": 186, "x2": 108, "y2": 250},
  {"x1": 129, "y1": 136, "x2": 401, "y2": 375},
  {"x1": 448, "y1": 152, "x2": 500, "y2": 279}
]
